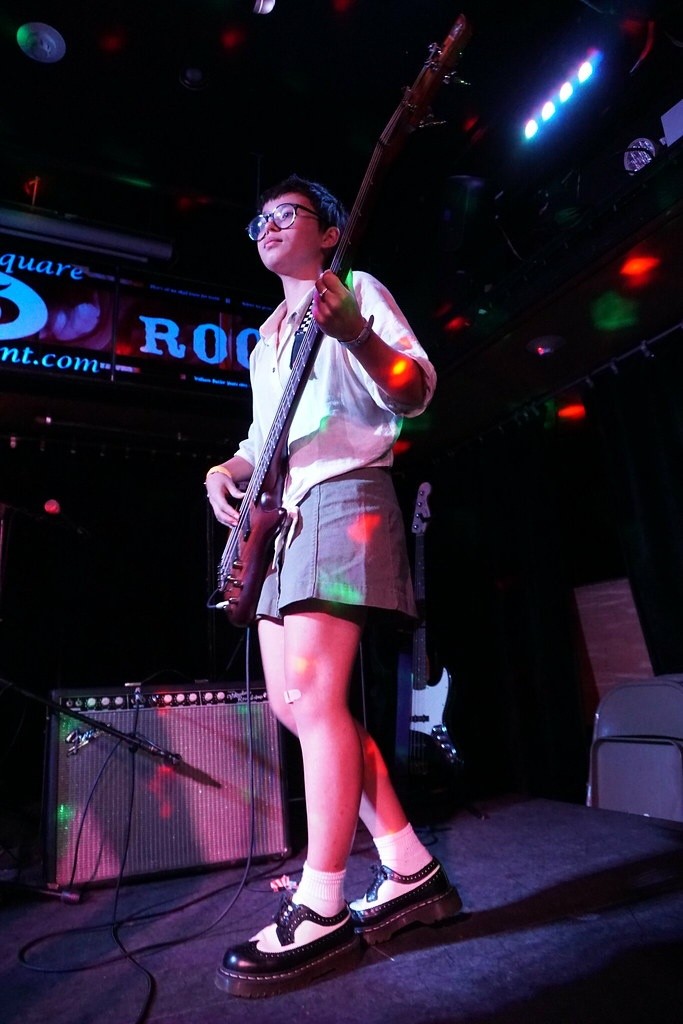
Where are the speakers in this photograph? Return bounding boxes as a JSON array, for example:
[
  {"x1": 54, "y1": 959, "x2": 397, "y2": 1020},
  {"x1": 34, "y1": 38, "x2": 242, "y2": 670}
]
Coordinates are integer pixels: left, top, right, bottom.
[{"x1": 48, "y1": 687, "x2": 295, "y2": 892}]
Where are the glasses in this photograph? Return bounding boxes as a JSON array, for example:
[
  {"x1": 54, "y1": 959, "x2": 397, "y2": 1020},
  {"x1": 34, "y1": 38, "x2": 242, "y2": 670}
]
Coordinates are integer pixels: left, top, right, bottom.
[{"x1": 244, "y1": 202, "x2": 324, "y2": 241}]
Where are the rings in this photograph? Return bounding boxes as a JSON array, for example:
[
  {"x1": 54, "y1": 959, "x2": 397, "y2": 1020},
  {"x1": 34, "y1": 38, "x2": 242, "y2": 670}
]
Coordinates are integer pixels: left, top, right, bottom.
[{"x1": 319, "y1": 288, "x2": 328, "y2": 298}]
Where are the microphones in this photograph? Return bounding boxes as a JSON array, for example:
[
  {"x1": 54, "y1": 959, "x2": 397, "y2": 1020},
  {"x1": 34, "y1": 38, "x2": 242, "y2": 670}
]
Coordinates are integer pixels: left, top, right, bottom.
[{"x1": 168, "y1": 753, "x2": 182, "y2": 766}]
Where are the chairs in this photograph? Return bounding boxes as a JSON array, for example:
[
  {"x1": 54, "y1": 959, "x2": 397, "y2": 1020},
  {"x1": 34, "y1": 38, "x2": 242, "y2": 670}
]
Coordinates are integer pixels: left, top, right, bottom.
[{"x1": 587, "y1": 674, "x2": 683, "y2": 822}]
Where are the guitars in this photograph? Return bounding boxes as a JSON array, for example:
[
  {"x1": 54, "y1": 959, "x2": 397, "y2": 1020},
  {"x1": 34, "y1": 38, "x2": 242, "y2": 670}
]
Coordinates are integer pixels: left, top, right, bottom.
[
  {"x1": 392, "y1": 480, "x2": 477, "y2": 788},
  {"x1": 215, "y1": 9, "x2": 471, "y2": 626}
]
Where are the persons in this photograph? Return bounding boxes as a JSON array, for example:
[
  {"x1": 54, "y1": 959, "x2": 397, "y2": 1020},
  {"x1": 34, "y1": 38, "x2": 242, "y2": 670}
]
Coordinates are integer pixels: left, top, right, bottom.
[{"x1": 204, "y1": 174, "x2": 464, "y2": 1000}]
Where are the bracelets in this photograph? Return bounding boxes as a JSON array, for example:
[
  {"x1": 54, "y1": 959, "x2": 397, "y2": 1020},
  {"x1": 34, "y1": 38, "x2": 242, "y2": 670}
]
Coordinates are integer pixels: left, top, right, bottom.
[
  {"x1": 337, "y1": 315, "x2": 374, "y2": 350},
  {"x1": 206, "y1": 466, "x2": 232, "y2": 479}
]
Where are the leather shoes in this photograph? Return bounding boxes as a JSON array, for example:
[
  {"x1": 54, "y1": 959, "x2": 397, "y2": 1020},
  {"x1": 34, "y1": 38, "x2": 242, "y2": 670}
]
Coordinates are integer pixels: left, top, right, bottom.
[
  {"x1": 215, "y1": 895, "x2": 362, "y2": 998},
  {"x1": 345, "y1": 861, "x2": 465, "y2": 945}
]
